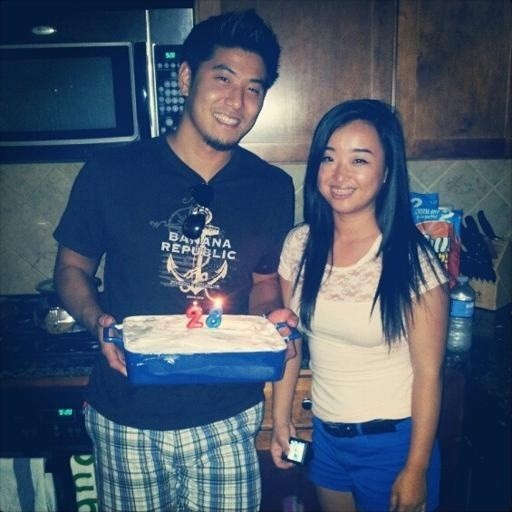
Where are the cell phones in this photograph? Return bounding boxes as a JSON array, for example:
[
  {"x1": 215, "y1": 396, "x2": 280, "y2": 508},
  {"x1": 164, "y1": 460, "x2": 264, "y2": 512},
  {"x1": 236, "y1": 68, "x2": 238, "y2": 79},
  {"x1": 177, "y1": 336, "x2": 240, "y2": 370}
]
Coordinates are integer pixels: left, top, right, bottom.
[{"x1": 282, "y1": 437, "x2": 311, "y2": 465}]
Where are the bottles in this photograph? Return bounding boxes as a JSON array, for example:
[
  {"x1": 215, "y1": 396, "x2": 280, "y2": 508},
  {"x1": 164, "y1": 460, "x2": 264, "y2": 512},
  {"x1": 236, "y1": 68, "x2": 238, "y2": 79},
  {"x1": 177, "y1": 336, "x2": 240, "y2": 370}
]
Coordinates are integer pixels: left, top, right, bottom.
[{"x1": 447, "y1": 272, "x2": 477, "y2": 355}]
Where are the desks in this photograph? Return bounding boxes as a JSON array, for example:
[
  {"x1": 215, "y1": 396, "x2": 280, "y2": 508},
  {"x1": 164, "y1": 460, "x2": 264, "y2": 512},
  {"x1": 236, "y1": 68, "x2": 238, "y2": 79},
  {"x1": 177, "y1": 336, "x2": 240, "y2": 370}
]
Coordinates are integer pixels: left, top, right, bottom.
[{"x1": 2, "y1": 293, "x2": 312, "y2": 511}]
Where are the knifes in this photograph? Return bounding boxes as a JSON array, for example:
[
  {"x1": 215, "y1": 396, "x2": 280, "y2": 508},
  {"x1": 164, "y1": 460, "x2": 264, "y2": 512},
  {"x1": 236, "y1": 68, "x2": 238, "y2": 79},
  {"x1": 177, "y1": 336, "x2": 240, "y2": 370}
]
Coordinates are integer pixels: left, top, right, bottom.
[{"x1": 458, "y1": 210, "x2": 497, "y2": 281}]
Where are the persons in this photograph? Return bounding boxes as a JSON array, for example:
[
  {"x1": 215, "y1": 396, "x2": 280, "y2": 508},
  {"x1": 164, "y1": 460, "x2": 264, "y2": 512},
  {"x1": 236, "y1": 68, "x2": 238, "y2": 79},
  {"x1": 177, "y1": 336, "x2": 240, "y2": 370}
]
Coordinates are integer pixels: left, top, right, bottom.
[
  {"x1": 51, "y1": 9, "x2": 301, "y2": 512},
  {"x1": 268, "y1": 98, "x2": 454, "y2": 512}
]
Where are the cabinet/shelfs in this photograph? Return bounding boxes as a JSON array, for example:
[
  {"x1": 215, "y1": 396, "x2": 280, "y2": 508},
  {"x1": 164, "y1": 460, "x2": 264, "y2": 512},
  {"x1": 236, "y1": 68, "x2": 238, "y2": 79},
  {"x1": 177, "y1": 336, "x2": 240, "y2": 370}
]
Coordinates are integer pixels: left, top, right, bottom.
[
  {"x1": 196, "y1": 2, "x2": 398, "y2": 167},
  {"x1": 398, "y1": 3, "x2": 512, "y2": 160}
]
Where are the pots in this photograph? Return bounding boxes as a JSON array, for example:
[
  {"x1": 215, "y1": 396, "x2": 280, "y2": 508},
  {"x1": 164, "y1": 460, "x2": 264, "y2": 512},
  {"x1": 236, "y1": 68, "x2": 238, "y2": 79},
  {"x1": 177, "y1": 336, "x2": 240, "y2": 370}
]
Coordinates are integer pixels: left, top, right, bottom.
[
  {"x1": 100, "y1": 310, "x2": 305, "y2": 387},
  {"x1": 31, "y1": 275, "x2": 103, "y2": 336}
]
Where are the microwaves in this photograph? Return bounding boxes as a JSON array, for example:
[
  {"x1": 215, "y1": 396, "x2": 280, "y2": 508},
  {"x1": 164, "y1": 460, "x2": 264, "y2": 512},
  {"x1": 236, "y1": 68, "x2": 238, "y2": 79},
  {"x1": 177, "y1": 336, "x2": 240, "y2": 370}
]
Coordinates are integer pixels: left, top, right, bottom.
[{"x1": 0, "y1": 2, "x2": 194, "y2": 166}]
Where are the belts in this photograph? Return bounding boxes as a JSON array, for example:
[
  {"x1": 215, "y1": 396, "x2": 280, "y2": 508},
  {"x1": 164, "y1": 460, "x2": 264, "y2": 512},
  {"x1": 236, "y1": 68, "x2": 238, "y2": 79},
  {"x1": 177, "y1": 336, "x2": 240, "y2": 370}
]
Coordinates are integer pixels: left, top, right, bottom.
[{"x1": 322, "y1": 418, "x2": 406, "y2": 439}]
